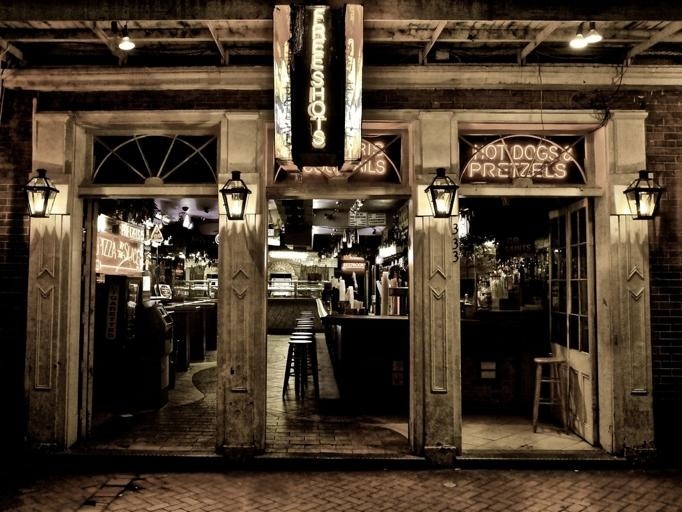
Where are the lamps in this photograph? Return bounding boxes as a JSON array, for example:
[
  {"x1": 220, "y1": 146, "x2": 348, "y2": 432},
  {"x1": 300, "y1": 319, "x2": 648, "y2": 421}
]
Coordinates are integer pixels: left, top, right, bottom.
[
  {"x1": 19, "y1": 161, "x2": 66, "y2": 222},
  {"x1": 218, "y1": 167, "x2": 256, "y2": 222},
  {"x1": 421, "y1": 165, "x2": 462, "y2": 220},
  {"x1": 567, "y1": 16, "x2": 607, "y2": 56},
  {"x1": 623, "y1": 166, "x2": 666, "y2": 223},
  {"x1": 109, "y1": 17, "x2": 141, "y2": 58}
]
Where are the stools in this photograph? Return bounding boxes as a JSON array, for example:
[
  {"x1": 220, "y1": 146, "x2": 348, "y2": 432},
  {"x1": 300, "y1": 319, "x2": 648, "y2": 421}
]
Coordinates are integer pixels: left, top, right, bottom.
[
  {"x1": 530, "y1": 350, "x2": 576, "y2": 439},
  {"x1": 283, "y1": 307, "x2": 329, "y2": 410}
]
